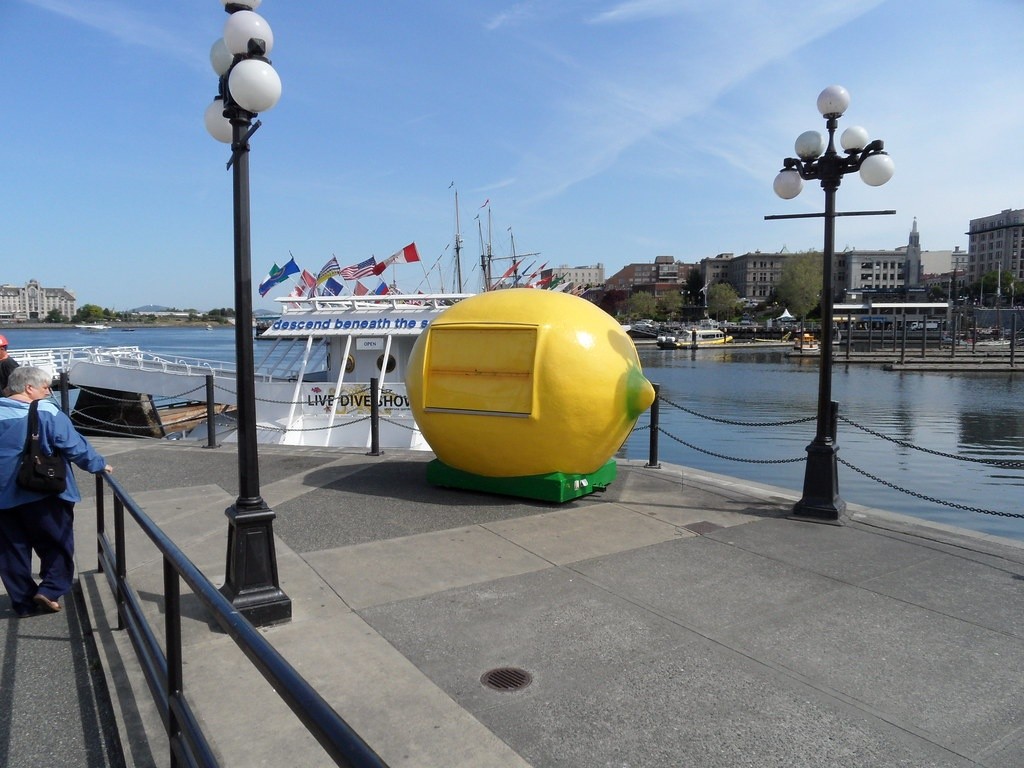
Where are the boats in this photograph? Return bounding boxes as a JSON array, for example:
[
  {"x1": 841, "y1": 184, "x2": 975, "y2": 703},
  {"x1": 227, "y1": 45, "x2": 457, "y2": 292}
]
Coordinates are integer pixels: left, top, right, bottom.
[
  {"x1": 658, "y1": 328, "x2": 734, "y2": 350},
  {"x1": 943, "y1": 334, "x2": 1024, "y2": 347},
  {"x1": 752, "y1": 332, "x2": 793, "y2": 343}
]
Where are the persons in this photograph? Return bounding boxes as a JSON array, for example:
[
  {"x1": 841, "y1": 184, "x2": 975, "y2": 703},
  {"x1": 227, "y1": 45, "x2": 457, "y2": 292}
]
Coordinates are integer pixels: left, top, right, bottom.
[
  {"x1": 0, "y1": 334, "x2": 20, "y2": 398},
  {"x1": 0, "y1": 366, "x2": 112, "y2": 618}
]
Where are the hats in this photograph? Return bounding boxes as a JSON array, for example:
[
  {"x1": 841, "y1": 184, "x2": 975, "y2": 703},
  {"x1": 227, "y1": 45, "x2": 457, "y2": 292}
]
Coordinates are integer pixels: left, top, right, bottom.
[{"x1": 0, "y1": 334, "x2": 8, "y2": 347}]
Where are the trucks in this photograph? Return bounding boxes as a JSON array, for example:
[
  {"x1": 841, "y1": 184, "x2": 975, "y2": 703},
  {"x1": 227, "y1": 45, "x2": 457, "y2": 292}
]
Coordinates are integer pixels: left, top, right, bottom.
[{"x1": 910, "y1": 320, "x2": 938, "y2": 332}]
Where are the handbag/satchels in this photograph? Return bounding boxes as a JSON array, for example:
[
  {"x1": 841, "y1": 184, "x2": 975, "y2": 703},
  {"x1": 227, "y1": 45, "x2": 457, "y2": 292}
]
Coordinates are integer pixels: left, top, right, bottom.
[{"x1": 16, "y1": 446, "x2": 68, "y2": 494}]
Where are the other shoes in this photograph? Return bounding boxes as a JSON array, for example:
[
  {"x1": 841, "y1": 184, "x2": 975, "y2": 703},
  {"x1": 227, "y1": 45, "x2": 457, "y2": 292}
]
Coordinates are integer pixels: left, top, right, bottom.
[
  {"x1": 16, "y1": 608, "x2": 33, "y2": 618},
  {"x1": 33, "y1": 594, "x2": 62, "y2": 612}
]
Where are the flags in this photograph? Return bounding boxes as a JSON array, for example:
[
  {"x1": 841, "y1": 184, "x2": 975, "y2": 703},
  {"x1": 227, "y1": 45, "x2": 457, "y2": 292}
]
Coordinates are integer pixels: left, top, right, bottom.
[
  {"x1": 259, "y1": 264, "x2": 289, "y2": 299},
  {"x1": 259, "y1": 258, "x2": 300, "y2": 293},
  {"x1": 307, "y1": 257, "x2": 341, "y2": 300},
  {"x1": 371, "y1": 243, "x2": 420, "y2": 276},
  {"x1": 340, "y1": 256, "x2": 376, "y2": 280},
  {"x1": 318, "y1": 277, "x2": 343, "y2": 308},
  {"x1": 287, "y1": 269, "x2": 317, "y2": 310},
  {"x1": 368, "y1": 281, "x2": 424, "y2": 305},
  {"x1": 353, "y1": 281, "x2": 369, "y2": 296},
  {"x1": 490, "y1": 258, "x2": 585, "y2": 296}
]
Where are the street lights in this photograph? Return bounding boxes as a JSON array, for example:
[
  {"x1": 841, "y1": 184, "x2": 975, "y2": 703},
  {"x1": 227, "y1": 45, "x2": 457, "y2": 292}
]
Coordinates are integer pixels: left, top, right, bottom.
[
  {"x1": 760, "y1": 86, "x2": 897, "y2": 536},
  {"x1": 204, "y1": 1, "x2": 293, "y2": 633}
]
